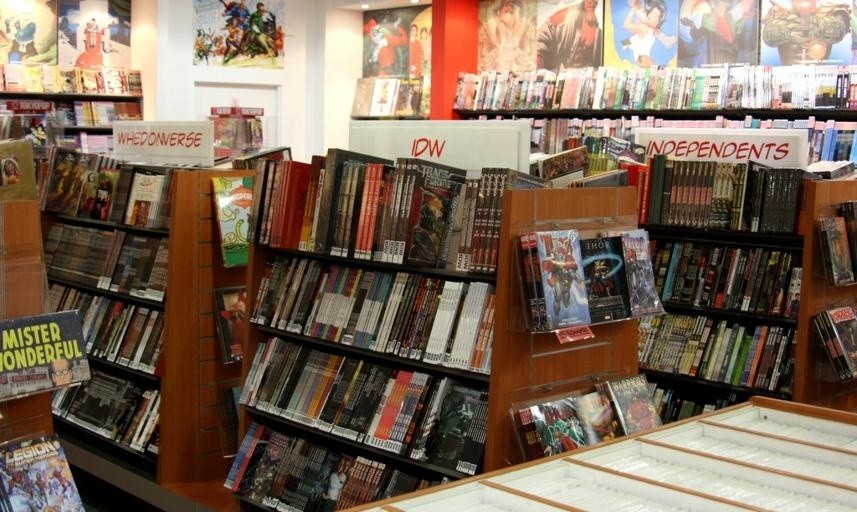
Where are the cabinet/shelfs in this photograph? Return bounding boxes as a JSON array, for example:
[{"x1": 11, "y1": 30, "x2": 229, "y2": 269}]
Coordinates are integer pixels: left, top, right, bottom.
[{"x1": 1, "y1": 89, "x2": 851, "y2": 512}]
